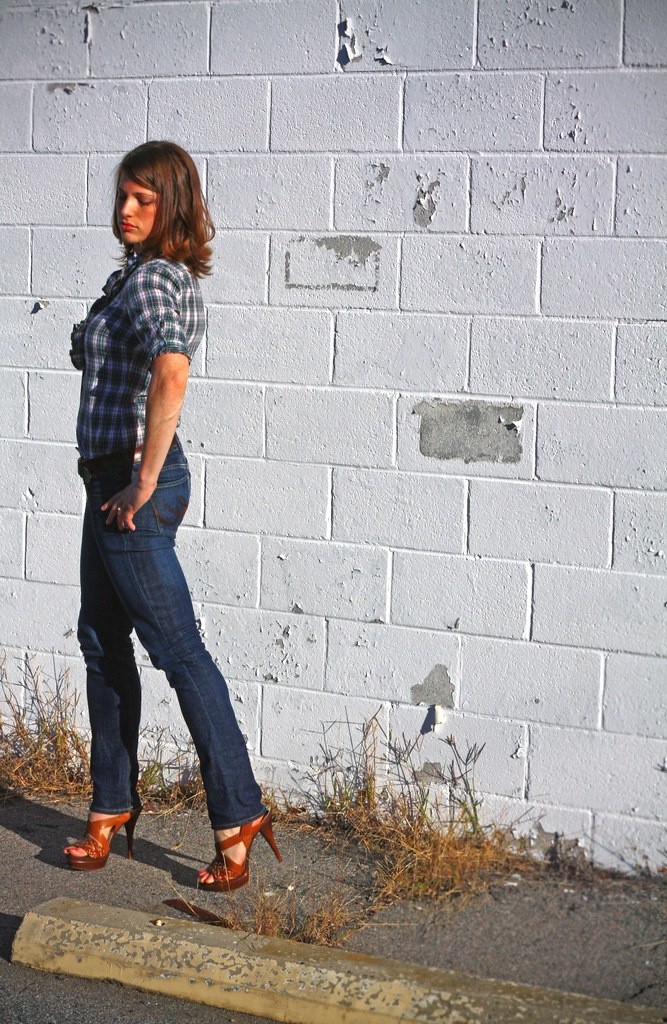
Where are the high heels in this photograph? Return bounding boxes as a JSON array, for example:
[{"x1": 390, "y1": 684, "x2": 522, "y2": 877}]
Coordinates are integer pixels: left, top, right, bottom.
[
  {"x1": 198, "y1": 808, "x2": 284, "y2": 894},
  {"x1": 63, "y1": 802, "x2": 145, "y2": 872}
]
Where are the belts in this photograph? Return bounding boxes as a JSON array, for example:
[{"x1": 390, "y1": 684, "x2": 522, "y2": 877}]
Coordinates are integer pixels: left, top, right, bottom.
[{"x1": 76, "y1": 438, "x2": 174, "y2": 485}]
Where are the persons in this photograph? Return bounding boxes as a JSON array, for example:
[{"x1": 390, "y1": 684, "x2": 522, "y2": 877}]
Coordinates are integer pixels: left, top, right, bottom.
[{"x1": 60, "y1": 140, "x2": 283, "y2": 893}]
